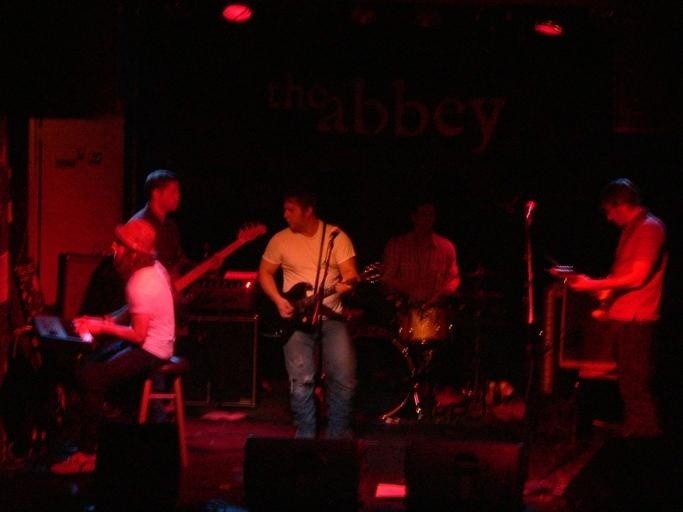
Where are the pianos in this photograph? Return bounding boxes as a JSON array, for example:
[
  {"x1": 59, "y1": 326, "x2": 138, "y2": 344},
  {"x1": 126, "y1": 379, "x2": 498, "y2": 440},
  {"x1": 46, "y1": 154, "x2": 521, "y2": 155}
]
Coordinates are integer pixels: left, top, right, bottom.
[{"x1": 30, "y1": 314, "x2": 100, "y2": 362}]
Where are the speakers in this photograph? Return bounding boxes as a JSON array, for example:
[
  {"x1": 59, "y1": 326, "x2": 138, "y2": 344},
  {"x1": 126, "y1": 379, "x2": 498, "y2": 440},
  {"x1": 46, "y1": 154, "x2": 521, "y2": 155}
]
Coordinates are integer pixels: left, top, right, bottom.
[
  {"x1": 404, "y1": 437, "x2": 527, "y2": 512},
  {"x1": 562, "y1": 436, "x2": 682, "y2": 510},
  {"x1": 91, "y1": 420, "x2": 179, "y2": 512},
  {"x1": 242, "y1": 437, "x2": 359, "y2": 512}
]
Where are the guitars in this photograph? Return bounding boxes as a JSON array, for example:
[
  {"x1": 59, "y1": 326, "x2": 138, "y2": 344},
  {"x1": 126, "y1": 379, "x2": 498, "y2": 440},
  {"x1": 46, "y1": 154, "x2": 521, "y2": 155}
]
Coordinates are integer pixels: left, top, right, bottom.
[
  {"x1": 258, "y1": 261, "x2": 385, "y2": 343},
  {"x1": 549, "y1": 265, "x2": 614, "y2": 324},
  {"x1": 167, "y1": 222, "x2": 267, "y2": 313}
]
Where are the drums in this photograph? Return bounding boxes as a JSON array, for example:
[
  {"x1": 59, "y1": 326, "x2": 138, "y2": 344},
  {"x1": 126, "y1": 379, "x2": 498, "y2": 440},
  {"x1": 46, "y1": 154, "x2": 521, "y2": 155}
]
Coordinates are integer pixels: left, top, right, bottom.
[
  {"x1": 396, "y1": 304, "x2": 453, "y2": 346},
  {"x1": 323, "y1": 326, "x2": 415, "y2": 423}
]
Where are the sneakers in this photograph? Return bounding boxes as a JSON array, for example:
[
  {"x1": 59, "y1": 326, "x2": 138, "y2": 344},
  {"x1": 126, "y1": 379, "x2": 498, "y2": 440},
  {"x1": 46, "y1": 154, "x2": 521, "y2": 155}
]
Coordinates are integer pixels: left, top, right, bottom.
[{"x1": 49, "y1": 449, "x2": 98, "y2": 475}]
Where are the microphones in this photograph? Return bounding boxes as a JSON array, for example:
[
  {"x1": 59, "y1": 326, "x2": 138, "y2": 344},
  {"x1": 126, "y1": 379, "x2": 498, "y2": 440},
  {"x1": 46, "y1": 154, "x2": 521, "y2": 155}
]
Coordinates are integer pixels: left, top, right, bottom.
[
  {"x1": 329, "y1": 226, "x2": 340, "y2": 237},
  {"x1": 524, "y1": 201, "x2": 536, "y2": 225}
]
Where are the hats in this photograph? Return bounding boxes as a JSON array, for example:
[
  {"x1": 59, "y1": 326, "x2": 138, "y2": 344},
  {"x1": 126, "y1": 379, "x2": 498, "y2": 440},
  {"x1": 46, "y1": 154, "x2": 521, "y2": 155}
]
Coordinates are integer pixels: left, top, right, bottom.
[{"x1": 113, "y1": 216, "x2": 157, "y2": 255}]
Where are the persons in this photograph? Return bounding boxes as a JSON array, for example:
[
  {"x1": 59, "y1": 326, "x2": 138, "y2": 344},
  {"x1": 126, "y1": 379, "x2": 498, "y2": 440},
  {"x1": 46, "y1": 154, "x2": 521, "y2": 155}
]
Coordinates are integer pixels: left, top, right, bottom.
[
  {"x1": 132, "y1": 172, "x2": 224, "y2": 337},
  {"x1": 49, "y1": 218, "x2": 174, "y2": 473},
  {"x1": 382, "y1": 201, "x2": 464, "y2": 310},
  {"x1": 257, "y1": 189, "x2": 360, "y2": 439},
  {"x1": 569, "y1": 178, "x2": 668, "y2": 436}
]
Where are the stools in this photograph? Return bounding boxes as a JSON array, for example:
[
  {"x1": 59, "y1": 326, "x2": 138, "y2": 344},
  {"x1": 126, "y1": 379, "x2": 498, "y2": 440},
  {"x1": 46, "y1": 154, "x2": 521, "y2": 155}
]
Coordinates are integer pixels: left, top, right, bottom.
[{"x1": 133, "y1": 354, "x2": 192, "y2": 469}]
